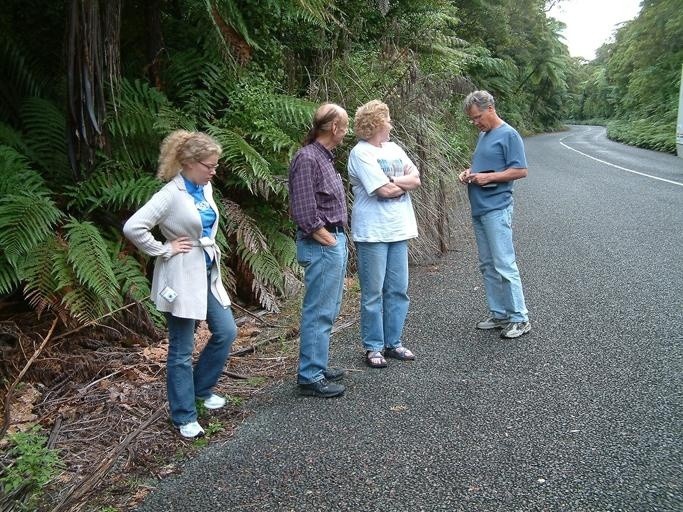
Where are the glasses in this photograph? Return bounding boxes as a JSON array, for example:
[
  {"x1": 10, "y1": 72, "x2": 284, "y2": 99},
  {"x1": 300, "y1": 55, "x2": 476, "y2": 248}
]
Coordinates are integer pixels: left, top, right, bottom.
[
  {"x1": 468, "y1": 116, "x2": 482, "y2": 125},
  {"x1": 198, "y1": 161, "x2": 219, "y2": 171}
]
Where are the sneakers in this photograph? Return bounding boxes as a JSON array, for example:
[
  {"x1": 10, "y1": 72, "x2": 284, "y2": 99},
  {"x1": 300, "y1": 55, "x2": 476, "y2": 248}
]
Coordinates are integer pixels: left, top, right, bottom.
[
  {"x1": 322, "y1": 366, "x2": 343, "y2": 381},
  {"x1": 476, "y1": 311, "x2": 510, "y2": 330},
  {"x1": 300, "y1": 378, "x2": 345, "y2": 397},
  {"x1": 499, "y1": 319, "x2": 531, "y2": 339},
  {"x1": 203, "y1": 394, "x2": 225, "y2": 409},
  {"x1": 179, "y1": 421, "x2": 205, "y2": 438}
]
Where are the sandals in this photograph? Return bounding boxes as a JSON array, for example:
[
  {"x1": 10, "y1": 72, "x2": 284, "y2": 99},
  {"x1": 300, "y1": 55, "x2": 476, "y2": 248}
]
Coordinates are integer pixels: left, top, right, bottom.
[
  {"x1": 366, "y1": 350, "x2": 387, "y2": 368},
  {"x1": 384, "y1": 346, "x2": 415, "y2": 360}
]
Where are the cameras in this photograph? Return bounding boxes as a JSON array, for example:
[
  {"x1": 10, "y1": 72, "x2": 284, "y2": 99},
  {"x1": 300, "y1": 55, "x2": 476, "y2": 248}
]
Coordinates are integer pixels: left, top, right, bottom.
[
  {"x1": 463, "y1": 174, "x2": 474, "y2": 183},
  {"x1": 159, "y1": 286, "x2": 178, "y2": 303}
]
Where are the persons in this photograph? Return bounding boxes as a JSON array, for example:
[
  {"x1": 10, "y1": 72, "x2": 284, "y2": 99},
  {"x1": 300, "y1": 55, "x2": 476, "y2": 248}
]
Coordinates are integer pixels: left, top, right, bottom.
[
  {"x1": 289, "y1": 103, "x2": 349, "y2": 398},
  {"x1": 122, "y1": 129, "x2": 237, "y2": 439},
  {"x1": 458, "y1": 90, "x2": 532, "y2": 340},
  {"x1": 347, "y1": 99, "x2": 421, "y2": 368}
]
